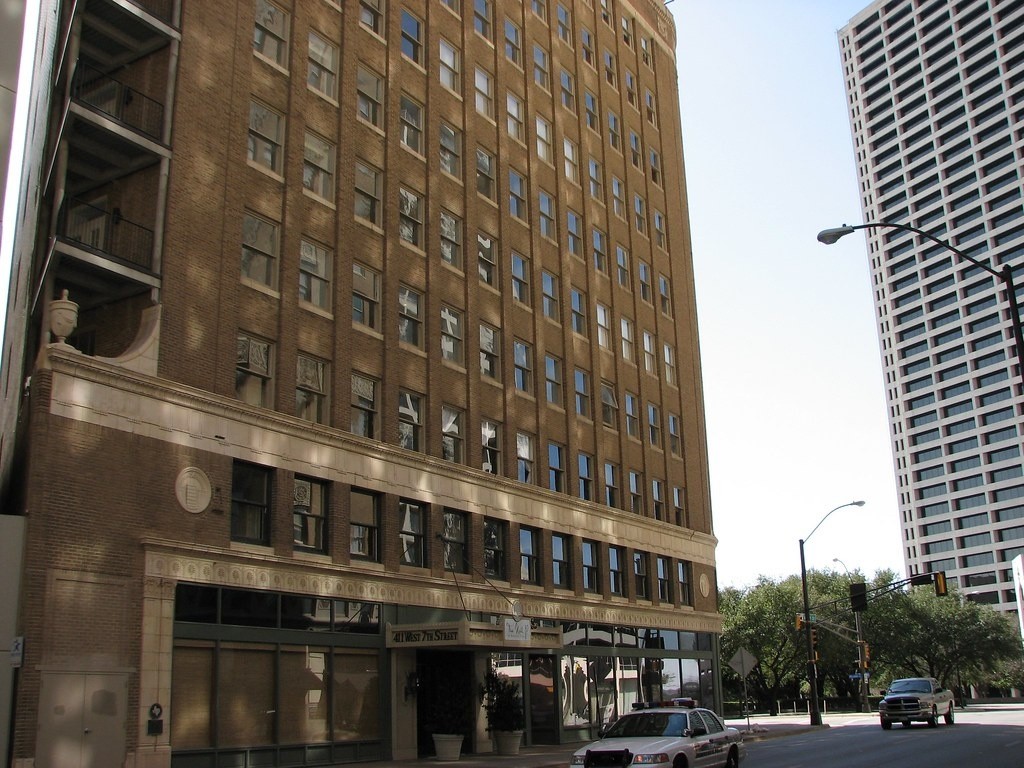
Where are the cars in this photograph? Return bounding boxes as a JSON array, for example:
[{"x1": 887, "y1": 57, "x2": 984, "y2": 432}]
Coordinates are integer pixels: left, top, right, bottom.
[{"x1": 570, "y1": 701, "x2": 747, "y2": 768}]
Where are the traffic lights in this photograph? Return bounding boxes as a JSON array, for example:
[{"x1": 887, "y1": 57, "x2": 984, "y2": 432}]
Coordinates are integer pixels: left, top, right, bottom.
[
  {"x1": 865, "y1": 660, "x2": 871, "y2": 668},
  {"x1": 811, "y1": 627, "x2": 818, "y2": 651},
  {"x1": 798, "y1": 615, "x2": 802, "y2": 630},
  {"x1": 865, "y1": 643, "x2": 869, "y2": 656}
]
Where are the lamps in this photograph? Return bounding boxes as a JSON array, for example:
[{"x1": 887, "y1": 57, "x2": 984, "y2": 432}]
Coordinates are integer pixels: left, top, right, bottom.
[
  {"x1": 404, "y1": 672, "x2": 424, "y2": 702},
  {"x1": 481, "y1": 668, "x2": 498, "y2": 700}
]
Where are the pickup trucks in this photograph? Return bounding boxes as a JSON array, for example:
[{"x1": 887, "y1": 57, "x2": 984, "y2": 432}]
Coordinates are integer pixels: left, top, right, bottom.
[{"x1": 878, "y1": 678, "x2": 955, "y2": 730}]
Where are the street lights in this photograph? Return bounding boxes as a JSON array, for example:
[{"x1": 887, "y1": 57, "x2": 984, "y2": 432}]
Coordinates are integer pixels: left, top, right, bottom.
[
  {"x1": 798, "y1": 501, "x2": 866, "y2": 725},
  {"x1": 833, "y1": 558, "x2": 854, "y2": 583},
  {"x1": 816, "y1": 223, "x2": 1023, "y2": 385}
]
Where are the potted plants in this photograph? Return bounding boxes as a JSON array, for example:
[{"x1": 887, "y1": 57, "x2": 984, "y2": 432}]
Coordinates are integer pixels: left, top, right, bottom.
[
  {"x1": 486, "y1": 667, "x2": 526, "y2": 756},
  {"x1": 428, "y1": 670, "x2": 475, "y2": 761}
]
